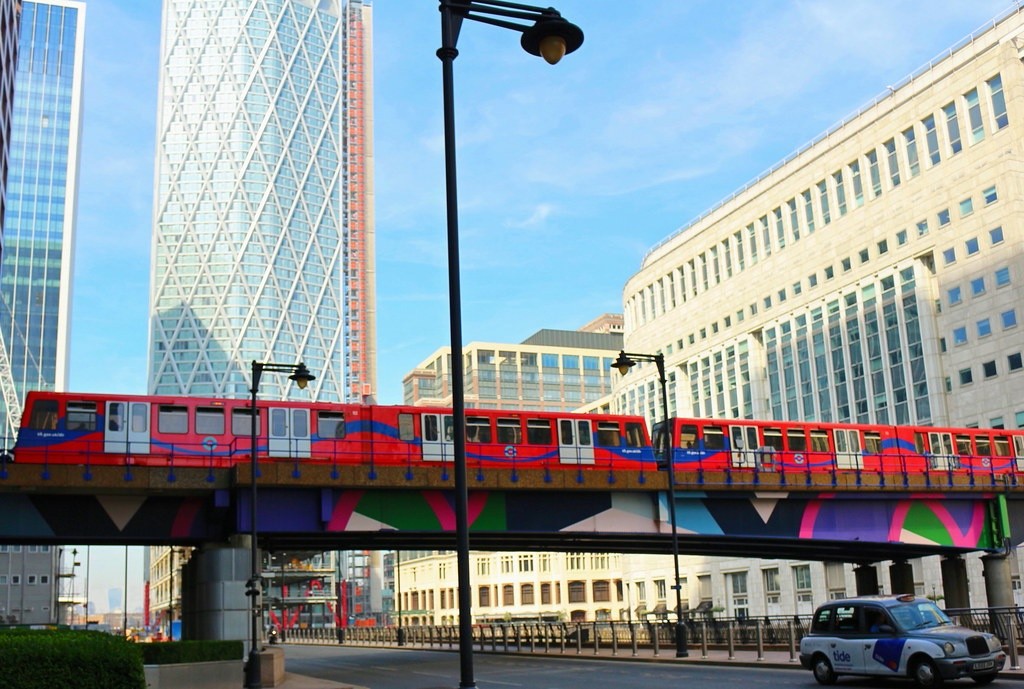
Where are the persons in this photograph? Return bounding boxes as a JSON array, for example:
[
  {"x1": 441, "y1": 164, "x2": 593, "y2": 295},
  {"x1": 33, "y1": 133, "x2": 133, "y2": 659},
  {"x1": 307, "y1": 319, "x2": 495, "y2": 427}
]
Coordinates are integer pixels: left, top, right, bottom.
[
  {"x1": 870, "y1": 612, "x2": 884, "y2": 635},
  {"x1": 268, "y1": 626, "x2": 277, "y2": 644}
]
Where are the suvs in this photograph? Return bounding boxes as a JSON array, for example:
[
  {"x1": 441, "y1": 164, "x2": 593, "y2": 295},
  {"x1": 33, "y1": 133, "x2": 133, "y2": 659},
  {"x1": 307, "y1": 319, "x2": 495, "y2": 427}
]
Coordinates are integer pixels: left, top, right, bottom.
[{"x1": 799, "y1": 593, "x2": 1007, "y2": 689}]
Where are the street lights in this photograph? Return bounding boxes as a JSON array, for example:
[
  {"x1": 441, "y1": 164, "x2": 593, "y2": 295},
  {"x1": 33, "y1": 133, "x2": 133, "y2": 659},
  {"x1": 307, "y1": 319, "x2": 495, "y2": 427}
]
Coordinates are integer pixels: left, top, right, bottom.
[
  {"x1": 433, "y1": 0, "x2": 585, "y2": 689},
  {"x1": 609, "y1": 348, "x2": 690, "y2": 658},
  {"x1": 244, "y1": 360, "x2": 317, "y2": 689}
]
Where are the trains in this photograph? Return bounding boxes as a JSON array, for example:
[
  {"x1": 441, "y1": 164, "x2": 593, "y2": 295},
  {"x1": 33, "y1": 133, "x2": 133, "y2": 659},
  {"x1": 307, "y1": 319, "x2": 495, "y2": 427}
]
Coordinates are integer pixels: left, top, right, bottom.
[{"x1": 12, "y1": 390, "x2": 1024, "y2": 476}]
